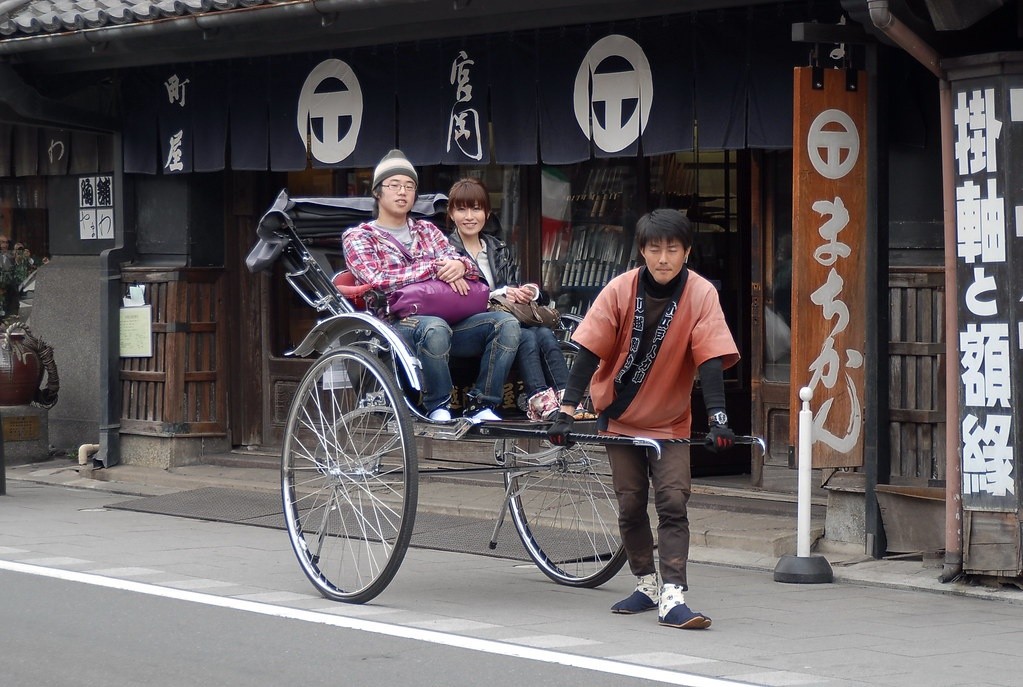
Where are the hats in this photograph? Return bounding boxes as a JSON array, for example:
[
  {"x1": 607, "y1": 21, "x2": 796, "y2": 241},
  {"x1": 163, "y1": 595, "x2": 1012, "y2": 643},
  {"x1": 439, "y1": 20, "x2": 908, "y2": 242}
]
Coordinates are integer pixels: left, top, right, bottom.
[{"x1": 372, "y1": 149, "x2": 418, "y2": 192}]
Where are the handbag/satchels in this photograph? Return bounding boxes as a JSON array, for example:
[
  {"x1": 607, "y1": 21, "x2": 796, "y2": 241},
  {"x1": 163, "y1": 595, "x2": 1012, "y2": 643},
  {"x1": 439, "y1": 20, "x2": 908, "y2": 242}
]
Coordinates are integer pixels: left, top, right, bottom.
[
  {"x1": 369, "y1": 226, "x2": 490, "y2": 321},
  {"x1": 487, "y1": 292, "x2": 559, "y2": 327}
]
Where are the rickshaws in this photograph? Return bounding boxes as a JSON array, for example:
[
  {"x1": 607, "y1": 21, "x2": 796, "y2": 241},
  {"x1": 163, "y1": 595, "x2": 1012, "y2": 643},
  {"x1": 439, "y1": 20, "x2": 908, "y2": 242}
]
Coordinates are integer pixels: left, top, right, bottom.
[{"x1": 244, "y1": 188, "x2": 769, "y2": 604}]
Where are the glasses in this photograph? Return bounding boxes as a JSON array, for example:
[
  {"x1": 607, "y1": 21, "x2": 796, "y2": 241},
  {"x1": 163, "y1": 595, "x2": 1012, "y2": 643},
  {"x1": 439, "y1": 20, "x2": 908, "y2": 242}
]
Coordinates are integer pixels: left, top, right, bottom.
[{"x1": 380, "y1": 182, "x2": 418, "y2": 191}]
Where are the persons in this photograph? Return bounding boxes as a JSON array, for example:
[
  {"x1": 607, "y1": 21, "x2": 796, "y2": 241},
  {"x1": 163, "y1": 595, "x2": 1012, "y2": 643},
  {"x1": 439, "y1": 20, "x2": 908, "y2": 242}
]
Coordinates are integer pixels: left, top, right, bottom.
[
  {"x1": 446, "y1": 178, "x2": 601, "y2": 422},
  {"x1": 341, "y1": 149, "x2": 522, "y2": 424},
  {"x1": 547, "y1": 209, "x2": 735, "y2": 629}
]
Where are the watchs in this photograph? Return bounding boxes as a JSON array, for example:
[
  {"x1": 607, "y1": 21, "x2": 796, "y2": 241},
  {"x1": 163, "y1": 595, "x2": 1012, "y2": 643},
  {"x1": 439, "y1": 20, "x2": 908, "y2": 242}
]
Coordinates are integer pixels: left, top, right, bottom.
[{"x1": 708, "y1": 411, "x2": 729, "y2": 426}]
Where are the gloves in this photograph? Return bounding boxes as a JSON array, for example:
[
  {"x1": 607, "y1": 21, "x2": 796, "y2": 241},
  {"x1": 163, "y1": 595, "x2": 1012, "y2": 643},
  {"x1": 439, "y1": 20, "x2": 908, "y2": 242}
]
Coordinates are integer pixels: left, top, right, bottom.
[
  {"x1": 705, "y1": 424, "x2": 735, "y2": 453},
  {"x1": 547, "y1": 412, "x2": 576, "y2": 449}
]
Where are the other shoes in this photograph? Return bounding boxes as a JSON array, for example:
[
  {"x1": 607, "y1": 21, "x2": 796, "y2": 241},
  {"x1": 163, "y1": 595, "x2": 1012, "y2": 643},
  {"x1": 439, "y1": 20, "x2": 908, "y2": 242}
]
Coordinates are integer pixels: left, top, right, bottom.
[
  {"x1": 610, "y1": 590, "x2": 659, "y2": 614},
  {"x1": 658, "y1": 604, "x2": 712, "y2": 630},
  {"x1": 429, "y1": 408, "x2": 452, "y2": 421},
  {"x1": 463, "y1": 401, "x2": 502, "y2": 420},
  {"x1": 574, "y1": 408, "x2": 598, "y2": 419}
]
